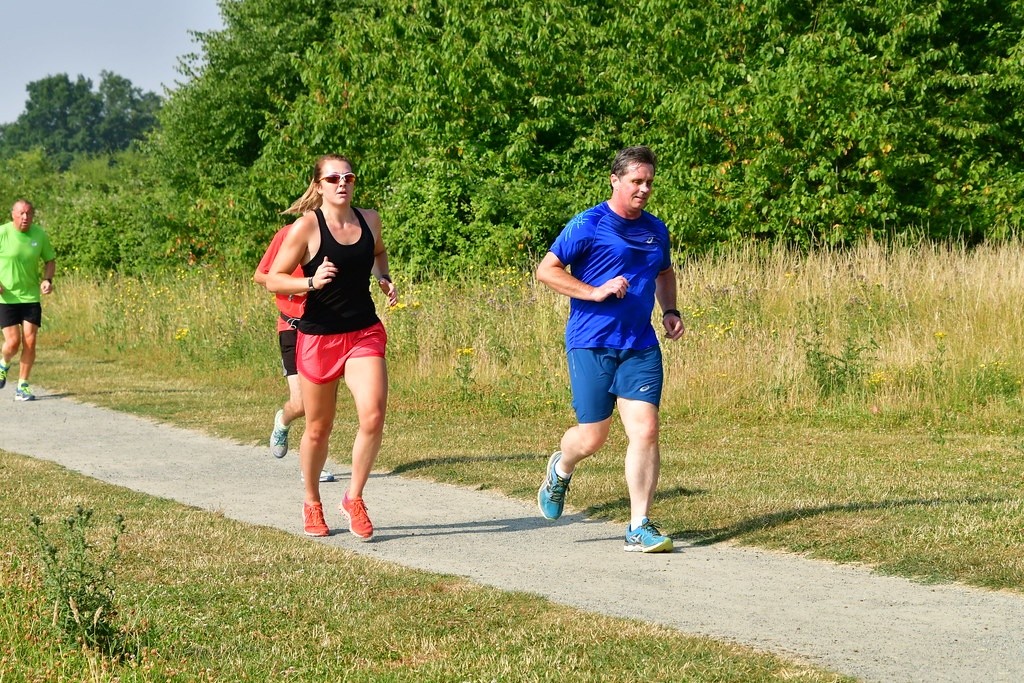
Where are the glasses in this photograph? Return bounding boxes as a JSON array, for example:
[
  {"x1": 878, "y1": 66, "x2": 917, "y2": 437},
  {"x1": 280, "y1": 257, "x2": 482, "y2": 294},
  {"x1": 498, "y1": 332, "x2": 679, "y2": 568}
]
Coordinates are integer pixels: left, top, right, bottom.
[{"x1": 317, "y1": 173, "x2": 356, "y2": 184}]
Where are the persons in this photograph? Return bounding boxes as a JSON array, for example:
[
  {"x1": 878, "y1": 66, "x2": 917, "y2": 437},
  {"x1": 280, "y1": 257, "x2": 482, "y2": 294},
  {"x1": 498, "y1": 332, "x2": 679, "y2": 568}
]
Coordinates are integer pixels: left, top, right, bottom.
[
  {"x1": 535, "y1": 147, "x2": 685, "y2": 553},
  {"x1": 265, "y1": 153, "x2": 398, "y2": 538},
  {"x1": 0, "y1": 199, "x2": 56, "y2": 401},
  {"x1": 253, "y1": 209, "x2": 335, "y2": 482}
]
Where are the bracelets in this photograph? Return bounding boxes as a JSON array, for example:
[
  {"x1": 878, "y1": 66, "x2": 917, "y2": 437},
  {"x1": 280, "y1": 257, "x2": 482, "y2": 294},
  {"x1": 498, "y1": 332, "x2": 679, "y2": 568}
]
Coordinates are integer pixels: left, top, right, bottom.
[
  {"x1": 662, "y1": 309, "x2": 681, "y2": 318},
  {"x1": 308, "y1": 277, "x2": 315, "y2": 291},
  {"x1": 378, "y1": 274, "x2": 392, "y2": 286},
  {"x1": 45, "y1": 278, "x2": 53, "y2": 284}
]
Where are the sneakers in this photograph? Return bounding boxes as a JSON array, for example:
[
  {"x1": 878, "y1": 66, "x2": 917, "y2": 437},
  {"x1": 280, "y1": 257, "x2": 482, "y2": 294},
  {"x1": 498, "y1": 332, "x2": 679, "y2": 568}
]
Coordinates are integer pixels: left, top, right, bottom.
[
  {"x1": 301, "y1": 468, "x2": 334, "y2": 482},
  {"x1": 623, "y1": 518, "x2": 673, "y2": 553},
  {"x1": 14, "y1": 383, "x2": 35, "y2": 400},
  {"x1": 0, "y1": 360, "x2": 12, "y2": 389},
  {"x1": 270, "y1": 410, "x2": 291, "y2": 458},
  {"x1": 537, "y1": 451, "x2": 575, "y2": 520},
  {"x1": 340, "y1": 489, "x2": 373, "y2": 541},
  {"x1": 301, "y1": 502, "x2": 329, "y2": 535}
]
